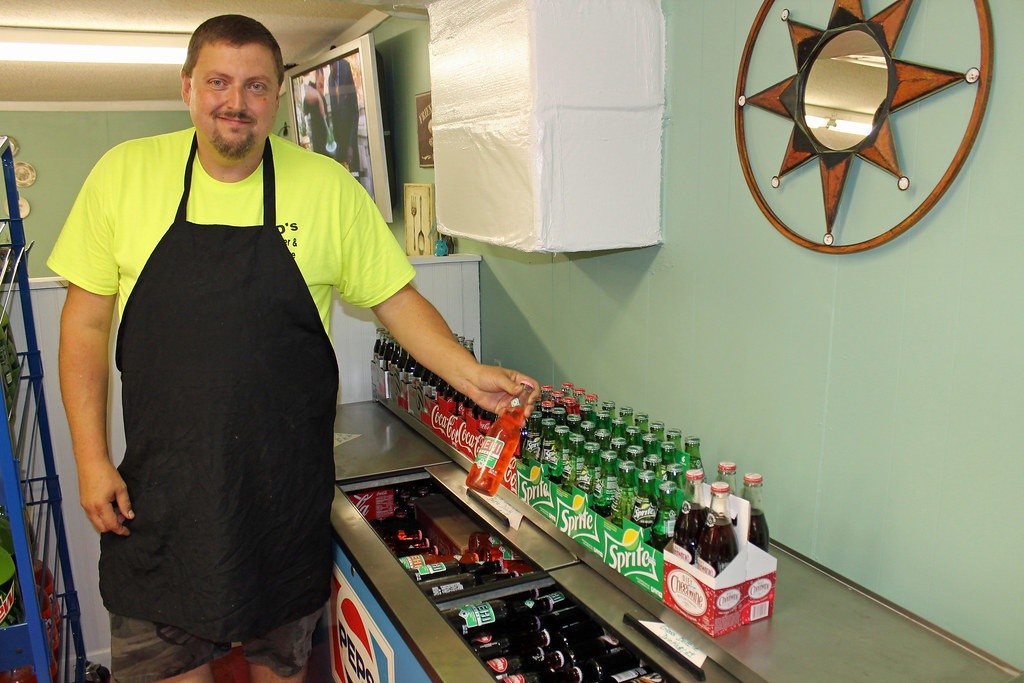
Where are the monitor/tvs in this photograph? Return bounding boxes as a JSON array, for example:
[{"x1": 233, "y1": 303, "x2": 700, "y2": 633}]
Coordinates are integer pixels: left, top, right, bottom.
[{"x1": 284, "y1": 34, "x2": 395, "y2": 224}]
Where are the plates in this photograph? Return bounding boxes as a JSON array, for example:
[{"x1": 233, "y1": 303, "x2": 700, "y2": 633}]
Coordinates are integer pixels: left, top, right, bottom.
[{"x1": 0, "y1": 134, "x2": 37, "y2": 219}]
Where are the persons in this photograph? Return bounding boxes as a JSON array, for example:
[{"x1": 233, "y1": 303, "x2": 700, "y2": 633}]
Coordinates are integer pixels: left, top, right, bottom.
[
  {"x1": 47, "y1": 14, "x2": 539, "y2": 683},
  {"x1": 329, "y1": 58, "x2": 361, "y2": 183},
  {"x1": 304, "y1": 68, "x2": 329, "y2": 159}
]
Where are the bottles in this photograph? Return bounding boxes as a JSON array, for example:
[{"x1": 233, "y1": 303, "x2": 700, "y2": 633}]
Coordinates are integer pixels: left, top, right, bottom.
[
  {"x1": 347, "y1": 327, "x2": 785, "y2": 682},
  {"x1": 0, "y1": 248, "x2": 73, "y2": 682}
]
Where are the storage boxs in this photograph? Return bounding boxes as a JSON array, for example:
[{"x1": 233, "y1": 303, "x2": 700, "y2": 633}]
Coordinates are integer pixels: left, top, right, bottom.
[{"x1": 370, "y1": 359, "x2": 777, "y2": 637}]
